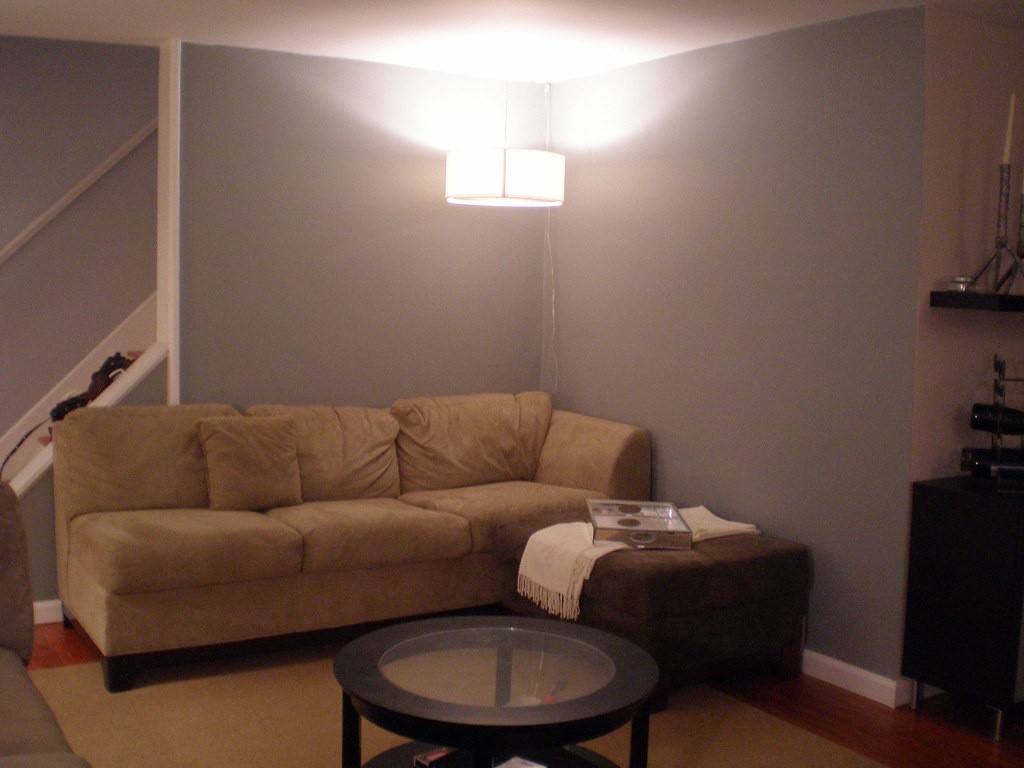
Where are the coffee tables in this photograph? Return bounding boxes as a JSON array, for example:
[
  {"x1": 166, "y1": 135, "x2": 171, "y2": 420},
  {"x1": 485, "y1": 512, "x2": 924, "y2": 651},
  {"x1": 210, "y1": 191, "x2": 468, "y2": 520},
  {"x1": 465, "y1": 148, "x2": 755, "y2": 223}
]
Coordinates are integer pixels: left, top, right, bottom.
[{"x1": 330, "y1": 618, "x2": 659, "y2": 768}]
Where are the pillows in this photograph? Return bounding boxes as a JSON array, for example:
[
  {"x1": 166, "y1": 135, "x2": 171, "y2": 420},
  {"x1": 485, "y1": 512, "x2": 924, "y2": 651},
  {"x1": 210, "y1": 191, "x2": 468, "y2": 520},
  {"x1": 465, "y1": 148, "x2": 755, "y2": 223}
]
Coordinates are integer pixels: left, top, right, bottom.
[
  {"x1": 195, "y1": 417, "x2": 302, "y2": 508},
  {"x1": 393, "y1": 392, "x2": 550, "y2": 495},
  {"x1": 253, "y1": 407, "x2": 400, "y2": 500}
]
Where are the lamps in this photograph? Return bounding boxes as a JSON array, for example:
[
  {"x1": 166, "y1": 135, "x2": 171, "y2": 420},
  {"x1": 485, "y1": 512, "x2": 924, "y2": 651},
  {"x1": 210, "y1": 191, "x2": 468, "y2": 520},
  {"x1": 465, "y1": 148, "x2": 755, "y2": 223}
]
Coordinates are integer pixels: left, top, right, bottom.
[{"x1": 444, "y1": 77, "x2": 568, "y2": 207}]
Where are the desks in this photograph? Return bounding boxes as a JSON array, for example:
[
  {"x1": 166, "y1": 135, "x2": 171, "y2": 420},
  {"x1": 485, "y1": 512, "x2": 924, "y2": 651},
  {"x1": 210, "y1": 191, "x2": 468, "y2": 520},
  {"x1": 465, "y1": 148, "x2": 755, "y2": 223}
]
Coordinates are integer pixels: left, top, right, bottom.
[{"x1": 902, "y1": 474, "x2": 1024, "y2": 744}]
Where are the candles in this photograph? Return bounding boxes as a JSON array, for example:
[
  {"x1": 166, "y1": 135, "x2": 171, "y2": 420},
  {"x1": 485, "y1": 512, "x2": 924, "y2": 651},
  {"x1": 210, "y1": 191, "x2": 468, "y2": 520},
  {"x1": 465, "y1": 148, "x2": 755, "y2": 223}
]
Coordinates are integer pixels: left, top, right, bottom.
[{"x1": 1003, "y1": 94, "x2": 1016, "y2": 166}]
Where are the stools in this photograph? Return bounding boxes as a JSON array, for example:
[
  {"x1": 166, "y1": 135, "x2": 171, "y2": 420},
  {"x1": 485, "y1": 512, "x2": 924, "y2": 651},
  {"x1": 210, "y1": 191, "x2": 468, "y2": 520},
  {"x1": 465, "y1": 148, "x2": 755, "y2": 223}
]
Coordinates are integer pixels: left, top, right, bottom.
[{"x1": 497, "y1": 514, "x2": 810, "y2": 722}]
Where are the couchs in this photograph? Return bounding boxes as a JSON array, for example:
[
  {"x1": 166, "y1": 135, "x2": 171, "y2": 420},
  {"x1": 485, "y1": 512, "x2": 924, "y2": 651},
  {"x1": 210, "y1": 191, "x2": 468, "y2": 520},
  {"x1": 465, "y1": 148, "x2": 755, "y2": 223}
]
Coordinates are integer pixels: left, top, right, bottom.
[{"x1": 52, "y1": 394, "x2": 650, "y2": 691}]
